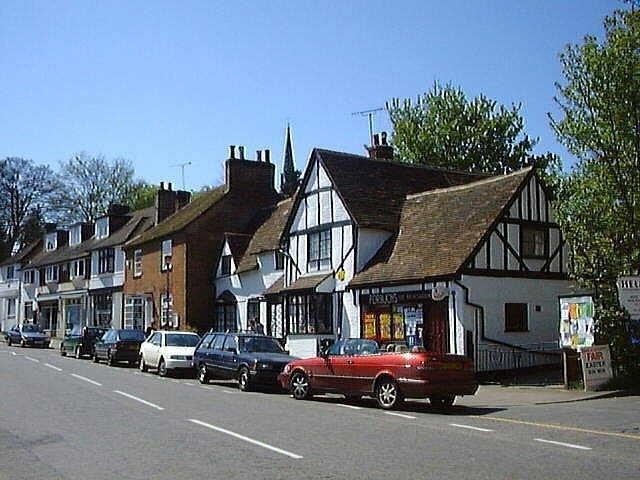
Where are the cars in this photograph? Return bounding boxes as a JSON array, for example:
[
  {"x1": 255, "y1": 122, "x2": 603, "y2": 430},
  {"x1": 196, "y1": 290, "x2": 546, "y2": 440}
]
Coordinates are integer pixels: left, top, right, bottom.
[
  {"x1": 60, "y1": 327, "x2": 302, "y2": 392},
  {"x1": 3, "y1": 324, "x2": 51, "y2": 348}
]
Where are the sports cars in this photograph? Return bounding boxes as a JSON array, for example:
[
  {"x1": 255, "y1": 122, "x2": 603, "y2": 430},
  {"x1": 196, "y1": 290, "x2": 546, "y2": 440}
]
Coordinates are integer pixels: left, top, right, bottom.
[{"x1": 277, "y1": 337, "x2": 478, "y2": 409}]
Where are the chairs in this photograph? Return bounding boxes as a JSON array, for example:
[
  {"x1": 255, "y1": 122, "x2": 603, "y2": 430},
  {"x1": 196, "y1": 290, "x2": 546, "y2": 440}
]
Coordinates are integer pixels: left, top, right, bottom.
[
  {"x1": 411, "y1": 345, "x2": 426, "y2": 352},
  {"x1": 385, "y1": 343, "x2": 394, "y2": 353},
  {"x1": 395, "y1": 344, "x2": 407, "y2": 353}
]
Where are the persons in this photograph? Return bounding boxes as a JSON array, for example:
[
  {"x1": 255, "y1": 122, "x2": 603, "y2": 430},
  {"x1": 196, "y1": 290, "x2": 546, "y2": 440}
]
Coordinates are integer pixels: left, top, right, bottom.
[
  {"x1": 246, "y1": 319, "x2": 255, "y2": 335},
  {"x1": 146, "y1": 322, "x2": 157, "y2": 334},
  {"x1": 253, "y1": 316, "x2": 265, "y2": 335}
]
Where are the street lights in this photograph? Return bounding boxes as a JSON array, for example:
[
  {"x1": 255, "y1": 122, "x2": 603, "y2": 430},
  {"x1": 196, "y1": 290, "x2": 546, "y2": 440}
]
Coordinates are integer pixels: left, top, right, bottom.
[{"x1": 164, "y1": 254, "x2": 173, "y2": 329}]
